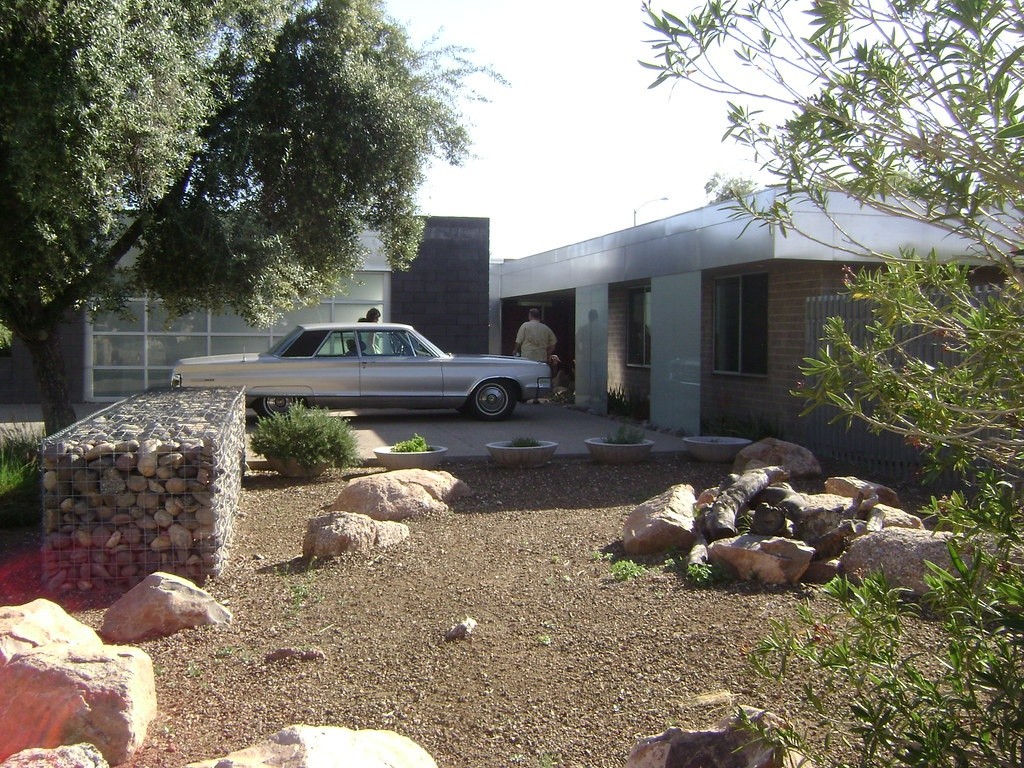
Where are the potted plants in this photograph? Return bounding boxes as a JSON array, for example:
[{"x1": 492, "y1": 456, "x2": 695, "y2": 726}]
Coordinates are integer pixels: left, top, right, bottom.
[
  {"x1": 374, "y1": 432, "x2": 448, "y2": 467},
  {"x1": 584, "y1": 416, "x2": 656, "y2": 463},
  {"x1": 682, "y1": 436, "x2": 752, "y2": 463},
  {"x1": 248, "y1": 396, "x2": 363, "y2": 477},
  {"x1": 485, "y1": 431, "x2": 560, "y2": 470}
]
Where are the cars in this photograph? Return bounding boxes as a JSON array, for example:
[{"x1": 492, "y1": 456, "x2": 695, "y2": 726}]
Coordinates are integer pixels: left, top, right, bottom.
[{"x1": 170, "y1": 322, "x2": 553, "y2": 421}]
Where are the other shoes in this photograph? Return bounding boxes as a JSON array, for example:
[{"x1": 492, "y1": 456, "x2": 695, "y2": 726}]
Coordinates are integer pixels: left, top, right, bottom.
[{"x1": 533, "y1": 399, "x2": 540, "y2": 405}]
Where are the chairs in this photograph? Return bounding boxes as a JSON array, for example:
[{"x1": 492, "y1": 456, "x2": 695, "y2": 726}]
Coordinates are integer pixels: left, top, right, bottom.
[
  {"x1": 359, "y1": 340, "x2": 369, "y2": 356},
  {"x1": 344, "y1": 340, "x2": 358, "y2": 357}
]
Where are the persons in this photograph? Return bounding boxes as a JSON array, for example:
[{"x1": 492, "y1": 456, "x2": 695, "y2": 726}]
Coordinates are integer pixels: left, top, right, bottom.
[
  {"x1": 359, "y1": 309, "x2": 381, "y2": 354},
  {"x1": 513, "y1": 309, "x2": 557, "y2": 404},
  {"x1": 576, "y1": 309, "x2": 607, "y2": 403}
]
